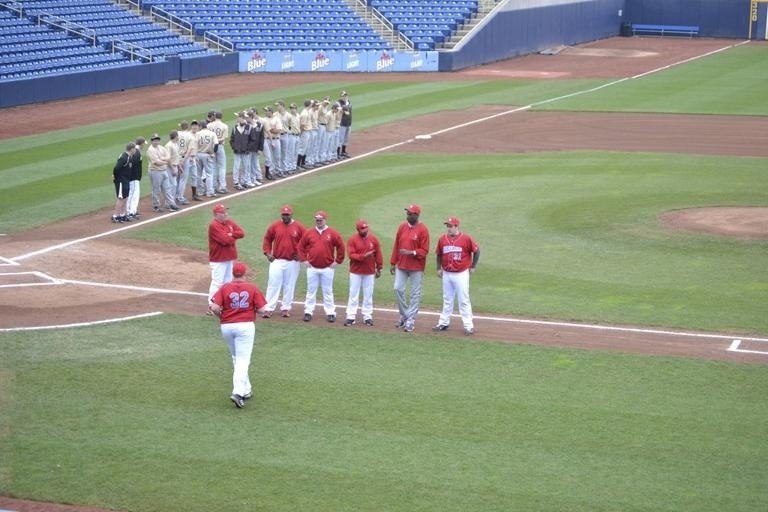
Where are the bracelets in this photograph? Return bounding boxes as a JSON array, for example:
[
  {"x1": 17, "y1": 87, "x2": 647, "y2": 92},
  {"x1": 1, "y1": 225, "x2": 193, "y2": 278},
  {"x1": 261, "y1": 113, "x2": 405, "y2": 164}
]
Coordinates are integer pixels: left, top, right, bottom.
[{"x1": 414, "y1": 250, "x2": 416, "y2": 256}]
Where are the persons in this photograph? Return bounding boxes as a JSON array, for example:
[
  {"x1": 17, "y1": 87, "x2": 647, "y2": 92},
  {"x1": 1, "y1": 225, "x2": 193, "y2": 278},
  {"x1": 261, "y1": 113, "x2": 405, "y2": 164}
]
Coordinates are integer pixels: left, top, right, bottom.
[
  {"x1": 344, "y1": 219, "x2": 383, "y2": 326},
  {"x1": 295, "y1": 210, "x2": 345, "y2": 322},
  {"x1": 211, "y1": 263, "x2": 268, "y2": 408},
  {"x1": 111, "y1": 92, "x2": 351, "y2": 222},
  {"x1": 206, "y1": 205, "x2": 245, "y2": 316},
  {"x1": 259, "y1": 205, "x2": 306, "y2": 317},
  {"x1": 433, "y1": 218, "x2": 479, "y2": 334},
  {"x1": 389, "y1": 203, "x2": 428, "y2": 331}
]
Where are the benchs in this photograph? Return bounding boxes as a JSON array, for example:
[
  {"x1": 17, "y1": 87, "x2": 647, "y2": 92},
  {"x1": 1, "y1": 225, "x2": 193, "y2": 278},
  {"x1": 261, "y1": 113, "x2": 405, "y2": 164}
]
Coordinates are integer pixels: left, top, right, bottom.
[{"x1": 632, "y1": 24, "x2": 700, "y2": 41}]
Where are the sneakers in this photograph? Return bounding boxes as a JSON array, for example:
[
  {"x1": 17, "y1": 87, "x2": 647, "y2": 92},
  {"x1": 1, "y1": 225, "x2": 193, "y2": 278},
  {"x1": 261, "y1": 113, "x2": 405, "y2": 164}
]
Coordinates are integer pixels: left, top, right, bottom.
[
  {"x1": 111, "y1": 214, "x2": 139, "y2": 223},
  {"x1": 152, "y1": 199, "x2": 189, "y2": 212},
  {"x1": 364, "y1": 320, "x2": 373, "y2": 326},
  {"x1": 328, "y1": 315, "x2": 335, "y2": 322},
  {"x1": 244, "y1": 392, "x2": 251, "y2": 399},
  {"x1": 234, "y1": 179, "x2": 262, "y2": 189},
  {"x1": 405, "y1": 325, "x2": 414, "y2": 332},
  {"x1": 191, "y1": 189, "x2": 227, "y2": 200},
  {"x1": 395, "y1": 321, "x2": 404, "y2": 328},
  {"x1": 281, "y1": 310, "x2": 289, "y2": 316},
  {"x1": 344, "y1": 320, "x2": 355, "y2": 327},
  {"x1": 262, "y1": 310, "x2": 272, "y2": 318},
  {"x1": 230, "y1": 395, "x2": 243, "y2": 407},
  {"x1": 431, "y1": 325, "x2": 448, "y2": 332},
  {"x1": 465, "y1": 329, "x2": 473, "y2": 336},
  {"x1": 304, "y1": 314, "x2": 311, "y2": 321},
  {"x1": 265, "y1": 153, "x2": 351, "y2": 179}
]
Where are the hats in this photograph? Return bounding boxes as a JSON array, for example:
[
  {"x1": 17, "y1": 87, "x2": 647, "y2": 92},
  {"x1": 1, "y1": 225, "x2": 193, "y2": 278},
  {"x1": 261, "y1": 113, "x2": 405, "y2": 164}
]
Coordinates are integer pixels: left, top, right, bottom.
[
  {"x1": 263, "y1": 101, "x2": 296, "y2": 111},
  {"x1": 234, "y1": 107, "x2": 258, "y2": 120},
  {"x1": 170, "y1": 110, "x2": 221, "y2": 139},
  {"x1": 315, "y1": 211, "x2": 326, "y2": 221},
  {"x1": 280, "y1": 206, "x2": 292, "y2": 215},
  {"x1": 233, "y1": 263, "x2": 246, "y2": 277},
  {"x1": 357, "y1": 221, "x2": 368, "y2": 233},
  {"x1": 213, "y1": 204, "x2": 228, "y2": 214},
  {"x1": 332, "y1": 91, "x2": 349, "y2": 111},
  {"x1": 404, "y1": 205, "x2": 420, "y2": 215},
  {"x1": 444, "y1": 218, "x2": 459, "y2": 226},
  {"x1": 304, "y1": 97, "x2": 330, "y2": 107},
  {"x1": 126, "y1": 134, "x2": 161, "y2": 149}
]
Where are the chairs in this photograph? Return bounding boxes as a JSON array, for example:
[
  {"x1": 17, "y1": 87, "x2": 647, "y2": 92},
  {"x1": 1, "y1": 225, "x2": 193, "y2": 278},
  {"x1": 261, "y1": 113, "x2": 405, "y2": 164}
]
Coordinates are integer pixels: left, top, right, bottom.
[{"x1": 1, "y1": 2, "x2": 479, "y2": 79}]
